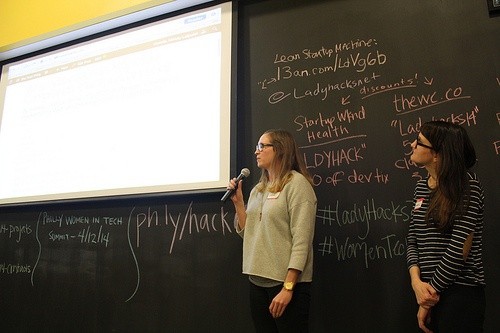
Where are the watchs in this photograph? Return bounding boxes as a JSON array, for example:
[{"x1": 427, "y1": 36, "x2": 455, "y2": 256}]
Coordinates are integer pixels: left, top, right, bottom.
[{"x1": 283, "y1": 282, "x2": 294, "y2": 291}]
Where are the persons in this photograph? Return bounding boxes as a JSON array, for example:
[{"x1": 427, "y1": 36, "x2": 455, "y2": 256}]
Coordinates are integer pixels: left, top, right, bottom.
[
  {"x1": 406, "y1": 121, "x2": 486, "y2": 333},
  {"x1": 227, "y1": 129, "x2": 317, "y2": 333}
]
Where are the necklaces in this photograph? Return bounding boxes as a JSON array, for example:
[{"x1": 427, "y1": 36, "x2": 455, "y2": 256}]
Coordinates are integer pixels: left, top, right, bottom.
[{"x1": 258, "y1": 190, "x2": 271, "y2": 222}]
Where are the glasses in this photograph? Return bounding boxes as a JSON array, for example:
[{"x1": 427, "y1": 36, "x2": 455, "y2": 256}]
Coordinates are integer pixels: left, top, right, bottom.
[
  {"x1": 256, "y1": 143, "x2": 273, "y2": 150},
  {"x1": 415, "y1": 135, "x2": 432, "y2": 149}
]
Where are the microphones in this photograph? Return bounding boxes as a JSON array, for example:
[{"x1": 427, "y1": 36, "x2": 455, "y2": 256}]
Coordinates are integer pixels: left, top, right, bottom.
[{"x1": 221, "y1": 168, "x2": 250, "y2": 203}]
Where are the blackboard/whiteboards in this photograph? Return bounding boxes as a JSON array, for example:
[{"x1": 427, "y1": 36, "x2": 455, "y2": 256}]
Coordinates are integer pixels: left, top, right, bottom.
[{"x1": 0, "y1": 2, "x2": 500, "y2": 332}]
